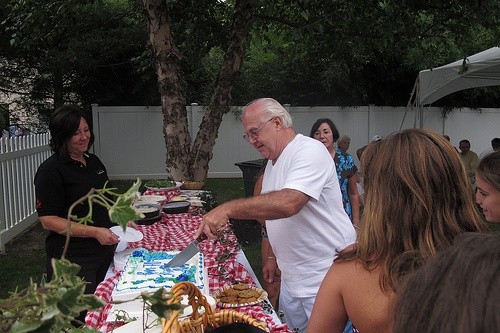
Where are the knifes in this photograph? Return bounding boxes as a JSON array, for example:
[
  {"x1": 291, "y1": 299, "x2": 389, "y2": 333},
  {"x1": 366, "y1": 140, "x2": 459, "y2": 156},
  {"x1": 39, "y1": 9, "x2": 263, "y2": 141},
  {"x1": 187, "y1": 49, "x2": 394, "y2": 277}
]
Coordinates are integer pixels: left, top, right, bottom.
[{"x1": 166, "y1": 232, "x2": 207, "y2": 267}]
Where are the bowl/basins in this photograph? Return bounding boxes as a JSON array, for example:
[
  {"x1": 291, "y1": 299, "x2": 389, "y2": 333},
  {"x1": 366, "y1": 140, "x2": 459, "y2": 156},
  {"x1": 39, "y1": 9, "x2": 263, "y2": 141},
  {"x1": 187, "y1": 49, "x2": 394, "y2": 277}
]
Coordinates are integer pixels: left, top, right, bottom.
[
  {"x1": 163, "y1": 201, "x2": 190, "y2": 213},
  {"x1": 134, "y1": 205, "x2": 160, "y2": 216}
]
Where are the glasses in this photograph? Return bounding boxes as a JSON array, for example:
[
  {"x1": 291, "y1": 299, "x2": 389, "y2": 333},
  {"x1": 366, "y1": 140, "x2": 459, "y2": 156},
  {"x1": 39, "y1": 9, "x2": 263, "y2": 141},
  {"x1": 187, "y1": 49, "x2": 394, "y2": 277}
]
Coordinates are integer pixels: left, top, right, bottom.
[{"x1": 242, "y1": 116, "x2": 274, "y2": 141}]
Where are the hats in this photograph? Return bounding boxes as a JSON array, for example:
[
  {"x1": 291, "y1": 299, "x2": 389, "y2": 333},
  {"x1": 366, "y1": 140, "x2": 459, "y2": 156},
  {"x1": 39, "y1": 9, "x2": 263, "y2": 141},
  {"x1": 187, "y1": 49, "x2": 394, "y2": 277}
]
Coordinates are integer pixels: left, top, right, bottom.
[{"x1": 372, "y1": 135, "x2": 381, "y2": 142}]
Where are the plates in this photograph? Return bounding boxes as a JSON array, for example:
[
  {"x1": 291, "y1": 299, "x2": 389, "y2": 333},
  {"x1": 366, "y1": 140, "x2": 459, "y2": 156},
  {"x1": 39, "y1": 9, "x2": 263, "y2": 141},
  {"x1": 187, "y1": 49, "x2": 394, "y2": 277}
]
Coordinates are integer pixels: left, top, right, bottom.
[
  {"x1": 110, "y1": 226, "x2": 143, "y2": 242},
  {"x1": 211, "y1": 285, "x2": 268, "y2": 308},
  {"x1": 141, "y1": 195, "x2": 167, "y2": 201},
  {"x1": 181, "y1": 182, "x2": 204, "y2": 188},
  {"x1": 144, "y1": 181, "x2": 182, "y2": 191}
]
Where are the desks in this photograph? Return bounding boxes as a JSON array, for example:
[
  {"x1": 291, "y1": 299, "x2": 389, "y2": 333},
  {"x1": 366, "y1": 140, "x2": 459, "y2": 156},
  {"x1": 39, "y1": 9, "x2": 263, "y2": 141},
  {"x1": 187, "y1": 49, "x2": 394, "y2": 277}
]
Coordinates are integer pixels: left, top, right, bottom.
[{"x1": 83, "y1": 189, "x2": 283, "y2": 333}]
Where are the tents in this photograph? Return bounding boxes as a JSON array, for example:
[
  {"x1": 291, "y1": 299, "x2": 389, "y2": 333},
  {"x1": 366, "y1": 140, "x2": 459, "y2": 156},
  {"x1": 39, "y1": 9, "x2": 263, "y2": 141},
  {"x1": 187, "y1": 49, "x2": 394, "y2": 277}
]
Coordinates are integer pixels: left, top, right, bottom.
[{"x1": 399, "y1": 46, "x2": 500, "y2": 131}]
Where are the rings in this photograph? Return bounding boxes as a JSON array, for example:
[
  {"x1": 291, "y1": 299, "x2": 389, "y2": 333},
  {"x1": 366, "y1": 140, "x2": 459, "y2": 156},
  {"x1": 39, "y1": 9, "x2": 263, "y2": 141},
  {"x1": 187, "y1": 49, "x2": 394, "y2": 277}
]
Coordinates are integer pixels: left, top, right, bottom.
[{"x1": 216, "y1": 229, "x2": 221, "y2": 231}]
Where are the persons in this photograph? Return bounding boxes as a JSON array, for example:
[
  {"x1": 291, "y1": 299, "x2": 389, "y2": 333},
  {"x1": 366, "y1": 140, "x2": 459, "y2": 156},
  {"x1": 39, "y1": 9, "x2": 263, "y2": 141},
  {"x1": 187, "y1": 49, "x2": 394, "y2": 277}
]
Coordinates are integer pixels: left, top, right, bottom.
[
  {"x1": 192, "y1": 97, "x2": 500, "y2": 333},
  {"x1": 32, "y1": 105, "x2": 138, "y2": 328}
]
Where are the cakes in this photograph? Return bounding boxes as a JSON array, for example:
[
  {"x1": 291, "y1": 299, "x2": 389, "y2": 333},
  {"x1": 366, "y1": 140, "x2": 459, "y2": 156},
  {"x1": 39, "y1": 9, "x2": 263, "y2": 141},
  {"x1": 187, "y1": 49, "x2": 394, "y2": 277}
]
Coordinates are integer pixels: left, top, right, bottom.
[{"x1": 110, "y1": 248, "x2": 207, "y2": 316}]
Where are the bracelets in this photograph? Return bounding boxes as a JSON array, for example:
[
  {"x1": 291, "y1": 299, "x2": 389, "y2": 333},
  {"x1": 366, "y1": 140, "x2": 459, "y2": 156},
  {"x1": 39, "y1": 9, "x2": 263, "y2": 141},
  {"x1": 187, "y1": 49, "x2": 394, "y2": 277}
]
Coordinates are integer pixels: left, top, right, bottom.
[{"x1": 267, "y1": 256, "x2": 276, "y2": 260}]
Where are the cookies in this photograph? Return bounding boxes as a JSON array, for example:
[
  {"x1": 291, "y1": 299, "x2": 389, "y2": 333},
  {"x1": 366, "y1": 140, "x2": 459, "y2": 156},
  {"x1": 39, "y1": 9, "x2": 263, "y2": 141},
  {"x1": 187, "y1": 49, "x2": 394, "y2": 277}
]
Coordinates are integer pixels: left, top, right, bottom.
[{"x1": 218, "y1": 283, "x2": 260, "y2": 303}]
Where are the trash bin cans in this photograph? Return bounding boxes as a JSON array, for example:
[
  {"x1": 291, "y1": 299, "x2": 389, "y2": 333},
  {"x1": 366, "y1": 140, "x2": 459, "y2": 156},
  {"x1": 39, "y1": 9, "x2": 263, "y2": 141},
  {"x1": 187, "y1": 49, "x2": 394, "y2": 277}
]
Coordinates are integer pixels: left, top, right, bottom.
[{"x1": 233, "y1": 158, "x2": 273, "y2": 245}]
